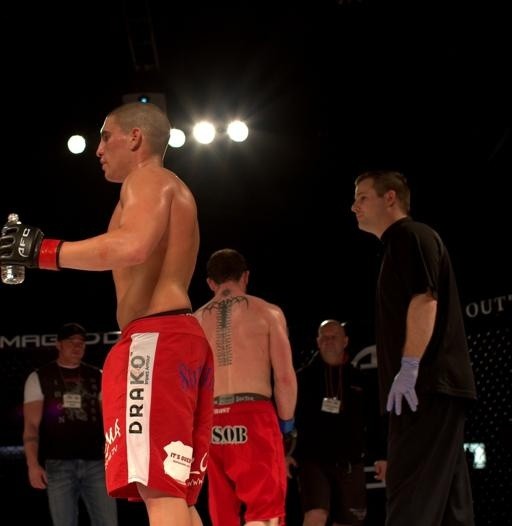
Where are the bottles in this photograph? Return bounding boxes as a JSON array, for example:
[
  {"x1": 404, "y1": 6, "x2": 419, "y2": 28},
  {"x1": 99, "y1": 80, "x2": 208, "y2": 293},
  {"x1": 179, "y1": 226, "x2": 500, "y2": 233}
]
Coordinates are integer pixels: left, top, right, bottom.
[{"x1": 1, "y1": 213, "x2": 26, "y2": 284}]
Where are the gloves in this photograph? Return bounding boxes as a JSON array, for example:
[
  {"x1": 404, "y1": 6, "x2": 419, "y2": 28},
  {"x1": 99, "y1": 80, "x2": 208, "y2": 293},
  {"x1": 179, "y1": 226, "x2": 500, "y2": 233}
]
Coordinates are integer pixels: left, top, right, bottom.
[
  {"x1": 1, "y1": 224, "x2": 63, "y2": 271},
  {"x1": 386, "y1": 356, "x2": 420, "y2": 415}
]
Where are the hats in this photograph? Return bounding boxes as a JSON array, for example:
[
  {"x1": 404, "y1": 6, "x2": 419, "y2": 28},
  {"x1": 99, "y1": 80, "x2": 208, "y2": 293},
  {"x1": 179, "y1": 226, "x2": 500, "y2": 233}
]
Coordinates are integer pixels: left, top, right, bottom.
[{"x1": 56, "y1": 324, "x2": 84, "y2": 341}]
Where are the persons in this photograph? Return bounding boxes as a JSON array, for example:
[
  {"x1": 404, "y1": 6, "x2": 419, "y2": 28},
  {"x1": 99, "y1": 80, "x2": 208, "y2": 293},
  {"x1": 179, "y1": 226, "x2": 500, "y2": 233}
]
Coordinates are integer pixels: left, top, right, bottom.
[
  {"x1": 294, "y1": 318, "x2": 388, "y2": 526},
  {"x1": 192, "y1": 248, "x2": 299, "y2": 526},
  {"x1": 351, "y1": 167, "x2": 480, "y2": 526},
  {"x1": 4, "y1": 102, "x2": 216, "y2": 526},
  {"x1": 23, "y1": 321, "x2": 120, "y2": 525}
]
